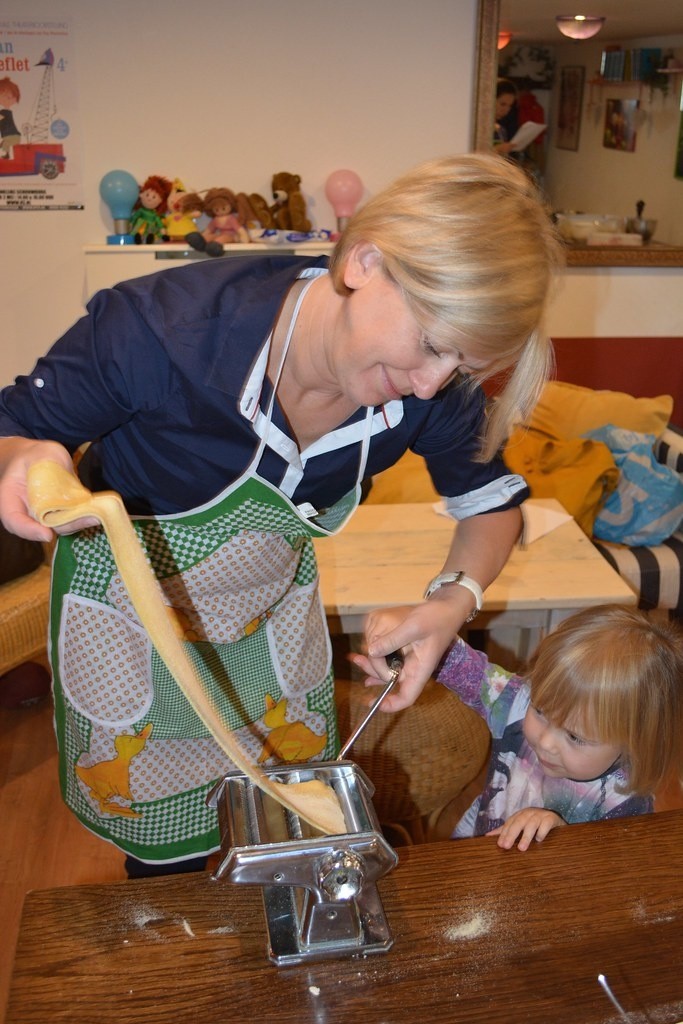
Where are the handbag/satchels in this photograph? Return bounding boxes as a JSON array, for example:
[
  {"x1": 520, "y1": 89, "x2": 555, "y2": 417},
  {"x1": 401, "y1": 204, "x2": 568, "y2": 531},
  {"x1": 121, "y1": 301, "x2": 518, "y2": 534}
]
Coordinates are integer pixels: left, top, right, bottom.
[{"x1": 510, "y1": 438, "x2": 622, "y2": 541}]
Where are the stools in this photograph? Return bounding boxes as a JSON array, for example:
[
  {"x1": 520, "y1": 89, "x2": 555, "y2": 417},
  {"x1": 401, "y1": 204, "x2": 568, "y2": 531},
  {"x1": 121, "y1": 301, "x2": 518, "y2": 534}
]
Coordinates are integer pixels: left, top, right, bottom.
[{"x1": 328, "y1": 670, "x2": 490, "y2": 848}]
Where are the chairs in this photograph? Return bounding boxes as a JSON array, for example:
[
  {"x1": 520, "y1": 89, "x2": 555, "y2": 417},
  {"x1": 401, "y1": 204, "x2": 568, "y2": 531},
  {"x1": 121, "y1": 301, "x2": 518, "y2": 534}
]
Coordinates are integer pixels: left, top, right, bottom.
[{"x1": 0, "y1": 521, "x2": 51, "y2": 682}]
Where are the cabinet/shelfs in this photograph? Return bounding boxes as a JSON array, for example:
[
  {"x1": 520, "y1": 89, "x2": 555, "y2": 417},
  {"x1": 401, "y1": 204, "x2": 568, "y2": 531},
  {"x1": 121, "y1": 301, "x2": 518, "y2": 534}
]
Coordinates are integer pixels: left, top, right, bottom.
[{"x1": 78, "y1": 239, "x2": 338, "y2": 319}]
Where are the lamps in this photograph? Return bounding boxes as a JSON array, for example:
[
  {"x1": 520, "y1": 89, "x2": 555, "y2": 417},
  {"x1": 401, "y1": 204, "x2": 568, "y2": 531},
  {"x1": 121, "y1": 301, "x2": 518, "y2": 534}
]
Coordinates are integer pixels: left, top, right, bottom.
[
  {"x1": 497, "y1": 31, "x2": 511, "y2": 50},
  {"x1": 556, "y1": 15, "x2": 605, "y2": 42}
]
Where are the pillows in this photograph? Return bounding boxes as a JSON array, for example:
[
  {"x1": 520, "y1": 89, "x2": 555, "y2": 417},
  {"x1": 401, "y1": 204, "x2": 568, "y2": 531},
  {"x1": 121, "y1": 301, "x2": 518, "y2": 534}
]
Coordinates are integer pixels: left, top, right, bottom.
[{"x1": 524, "y1": 379, "x2": 673, "y2": 445}]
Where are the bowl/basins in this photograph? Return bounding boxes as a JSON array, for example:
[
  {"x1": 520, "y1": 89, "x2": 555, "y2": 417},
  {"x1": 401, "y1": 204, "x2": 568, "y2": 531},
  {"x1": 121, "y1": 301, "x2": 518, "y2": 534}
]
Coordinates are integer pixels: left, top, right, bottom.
[{"x1": 626, "y1": 218, "x2": 657, "y2": 246}]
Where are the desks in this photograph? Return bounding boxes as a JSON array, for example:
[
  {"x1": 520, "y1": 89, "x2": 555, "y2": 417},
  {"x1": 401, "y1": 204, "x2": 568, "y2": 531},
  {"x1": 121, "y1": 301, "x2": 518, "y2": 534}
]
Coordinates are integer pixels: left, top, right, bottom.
[
  {"x1": 311, "y1": 498, "x2": 638, "y2": 665},
  {"x1": 0, "y1": 808, "x2": 683, "y2": 1024}
]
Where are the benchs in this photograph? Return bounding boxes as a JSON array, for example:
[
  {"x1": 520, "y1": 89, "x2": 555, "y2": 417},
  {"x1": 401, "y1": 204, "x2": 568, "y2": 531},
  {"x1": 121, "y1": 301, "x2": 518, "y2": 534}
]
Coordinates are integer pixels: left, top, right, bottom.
[{"x1": 359, "y1": 417, "x2": 683, "y2": 625}]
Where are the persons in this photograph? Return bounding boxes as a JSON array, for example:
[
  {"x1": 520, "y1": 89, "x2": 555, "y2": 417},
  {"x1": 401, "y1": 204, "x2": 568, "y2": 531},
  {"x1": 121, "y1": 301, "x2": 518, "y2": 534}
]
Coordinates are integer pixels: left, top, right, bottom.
[
  {"x1": 492, "y1": 77, "x2": 516, "y2": 156},
  {"x1": 0, "y1": 152, "x2": 566, "y2": 879},
  {"x1": 361, "y1": 606, "x2": 682, "y2": 851}
]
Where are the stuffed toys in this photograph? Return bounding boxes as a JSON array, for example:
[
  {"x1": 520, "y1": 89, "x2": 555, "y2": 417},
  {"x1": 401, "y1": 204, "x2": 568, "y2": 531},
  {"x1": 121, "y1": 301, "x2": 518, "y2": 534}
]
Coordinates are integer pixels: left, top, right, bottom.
[
  {"x1": 161, "y1": 178, "x2": 201, "y2": 242},
  {"x1": 235, "y1": 193, "x2": 276, "y2": 230},
  {"x1": 185, "y1": 188, "x2": 250, "y2": 255},
  {"x1": 128, "y1": 176, "x2": 174, "y2": 245},
  {"x1": 269, "y1": 172, "x2": 311, "y2": 233}
]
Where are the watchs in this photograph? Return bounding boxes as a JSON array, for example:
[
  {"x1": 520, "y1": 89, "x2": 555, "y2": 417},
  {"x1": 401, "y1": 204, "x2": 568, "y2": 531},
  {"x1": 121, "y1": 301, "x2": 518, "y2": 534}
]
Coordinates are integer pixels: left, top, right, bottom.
[{"x1": 423, "y1": 570, "x2": 483, "y2": 624}]
